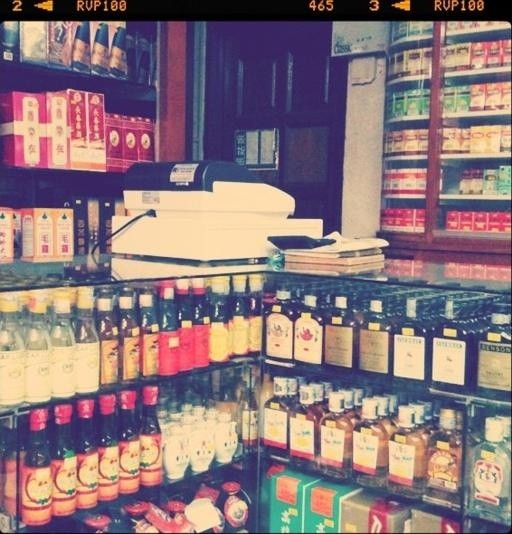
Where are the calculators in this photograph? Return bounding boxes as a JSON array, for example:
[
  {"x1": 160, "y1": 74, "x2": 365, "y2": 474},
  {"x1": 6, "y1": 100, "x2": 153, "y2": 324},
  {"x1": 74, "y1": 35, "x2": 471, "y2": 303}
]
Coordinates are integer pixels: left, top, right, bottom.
[{"x1": 268, "y1": 236, "x2": 336, "y2": 249}]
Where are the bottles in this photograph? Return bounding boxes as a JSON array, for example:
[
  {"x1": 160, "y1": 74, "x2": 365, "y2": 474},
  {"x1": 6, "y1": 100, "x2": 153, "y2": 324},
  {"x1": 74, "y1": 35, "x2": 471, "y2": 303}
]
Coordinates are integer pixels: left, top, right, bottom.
[
  {"x1": 256, "y1": 283, "x2": 512, "y2": 526},
  {"x1": 1, "y1": 22, "x2": 127, "y2": 78},
  {"x1": 1, "y1": 273, "x2": 262, "y2": 533}
]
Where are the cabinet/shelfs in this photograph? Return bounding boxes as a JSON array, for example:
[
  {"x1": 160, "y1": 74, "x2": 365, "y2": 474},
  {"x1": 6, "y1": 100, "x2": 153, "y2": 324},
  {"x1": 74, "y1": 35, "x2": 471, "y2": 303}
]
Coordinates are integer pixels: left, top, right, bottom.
[{"x1": 0, "y1": 17, "x2": 511, "y2": 534}]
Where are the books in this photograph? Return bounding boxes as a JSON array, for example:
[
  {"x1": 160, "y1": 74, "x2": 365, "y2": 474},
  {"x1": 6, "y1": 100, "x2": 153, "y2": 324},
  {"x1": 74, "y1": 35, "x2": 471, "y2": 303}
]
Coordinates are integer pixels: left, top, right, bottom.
[{"x1": 285, "y1": 229, "x2": 390, "y2": 264}]
